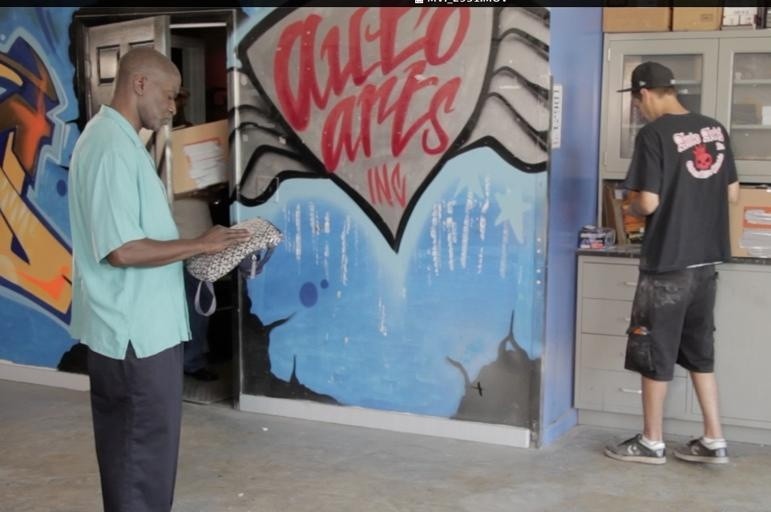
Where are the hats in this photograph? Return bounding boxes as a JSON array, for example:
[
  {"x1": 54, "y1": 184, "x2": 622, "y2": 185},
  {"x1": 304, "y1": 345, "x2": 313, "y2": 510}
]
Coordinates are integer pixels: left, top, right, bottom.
[{"x1": 616, "y1": 60, "x2": 677, "y2": 93}]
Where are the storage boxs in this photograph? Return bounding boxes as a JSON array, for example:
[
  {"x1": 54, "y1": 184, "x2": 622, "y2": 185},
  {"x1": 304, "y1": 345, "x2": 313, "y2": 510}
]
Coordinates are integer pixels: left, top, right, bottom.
[
  {"x1": 600, "y1": 7, "x2": 721, "y2": 33},
  {"x1": 167, "y1": 119, "x2": 233, "y2": 193},
  {"x1": 725, "y1": 182, "x2": 771, "y2": 257}
]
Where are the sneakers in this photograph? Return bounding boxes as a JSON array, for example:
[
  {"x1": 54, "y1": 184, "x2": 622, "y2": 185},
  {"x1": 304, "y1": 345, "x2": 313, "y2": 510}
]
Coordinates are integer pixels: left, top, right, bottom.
[
  {"x1": 603, "y1": 433, "x2": 667, "y2": 465},
  {"x1": 672, "y1": 437, "x2": 730, "y2": 464}
]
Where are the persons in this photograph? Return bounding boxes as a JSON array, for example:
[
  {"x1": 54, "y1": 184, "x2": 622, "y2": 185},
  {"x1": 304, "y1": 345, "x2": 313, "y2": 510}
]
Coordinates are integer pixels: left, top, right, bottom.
[
  {"x1": 603, "y1": 62, "x2": 739, "y2": 464},
  {"x1": 67, "y1": 47, "x2": 250, "y2": 512}
]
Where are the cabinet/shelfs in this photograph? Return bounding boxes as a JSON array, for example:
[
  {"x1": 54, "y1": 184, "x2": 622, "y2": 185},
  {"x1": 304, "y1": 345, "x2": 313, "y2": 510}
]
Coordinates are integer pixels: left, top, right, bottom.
[
  {"x1": 602, "y1": 36, "x2": 771, "y2": 179},
  {"x1": 688, "y1": 262, "x2": 770, "y2": 429},
  {"x1": 568, "y1": 253, "x2": 689, "y2": 421}
]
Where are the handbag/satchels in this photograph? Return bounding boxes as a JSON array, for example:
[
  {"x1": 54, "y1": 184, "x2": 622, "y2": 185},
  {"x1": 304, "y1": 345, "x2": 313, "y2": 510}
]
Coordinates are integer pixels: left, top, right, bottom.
[{"x1": 185, "y1": 216, "x2": 284, "y2": 284}]
